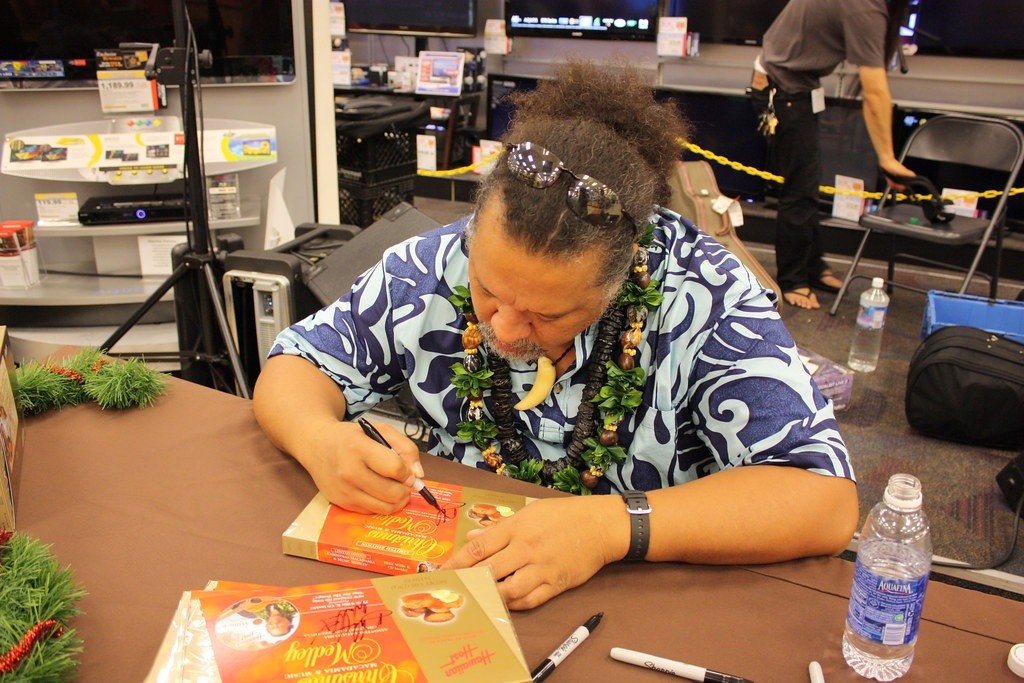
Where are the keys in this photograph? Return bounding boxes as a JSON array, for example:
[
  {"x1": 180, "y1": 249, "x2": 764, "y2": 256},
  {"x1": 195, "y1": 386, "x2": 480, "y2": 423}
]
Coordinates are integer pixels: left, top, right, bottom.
[{"x1": 756, "y1": 89, "x2": 780, "y2": 136}]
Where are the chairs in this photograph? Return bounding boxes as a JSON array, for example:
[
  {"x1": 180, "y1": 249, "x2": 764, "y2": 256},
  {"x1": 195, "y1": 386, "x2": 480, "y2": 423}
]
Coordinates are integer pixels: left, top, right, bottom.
[{"x1": 828, "y1": 112, "x2": 1022, "y2": 317}]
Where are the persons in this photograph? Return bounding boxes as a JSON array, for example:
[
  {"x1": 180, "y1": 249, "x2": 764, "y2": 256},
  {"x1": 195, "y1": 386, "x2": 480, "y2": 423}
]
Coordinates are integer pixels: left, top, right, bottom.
[
  {"x1": 252, "y1": 57, "x2": 860, "y2": 615},
  {"x1": 749, "y1": 0, "x2": 916, "y2": 308}
]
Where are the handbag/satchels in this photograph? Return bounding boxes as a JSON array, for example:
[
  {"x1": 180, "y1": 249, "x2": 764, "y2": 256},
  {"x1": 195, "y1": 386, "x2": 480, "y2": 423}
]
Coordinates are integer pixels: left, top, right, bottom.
[{"x1": 905, "y1": 326, "x2": 1024, "y2": 452}]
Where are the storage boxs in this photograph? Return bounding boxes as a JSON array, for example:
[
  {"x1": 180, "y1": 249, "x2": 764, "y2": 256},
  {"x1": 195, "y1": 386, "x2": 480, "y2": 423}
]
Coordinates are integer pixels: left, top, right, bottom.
[
  {"x1": 334, "y1": 114, "x2": 446, "y2": 227},
  {"x1": 921, "y1": 287, "x2": 1024, "y2": 340}
]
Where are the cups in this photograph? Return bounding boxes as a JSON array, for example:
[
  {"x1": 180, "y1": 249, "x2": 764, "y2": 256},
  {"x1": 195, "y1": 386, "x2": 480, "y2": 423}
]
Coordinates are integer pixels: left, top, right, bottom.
[{"x1": 369, "y1": 66, "x2": 387, "y2": 85}]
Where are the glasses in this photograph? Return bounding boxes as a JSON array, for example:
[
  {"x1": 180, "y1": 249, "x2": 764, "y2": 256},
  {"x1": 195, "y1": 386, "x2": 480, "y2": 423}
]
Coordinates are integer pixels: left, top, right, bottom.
[{"x1": 505, "y1": 141, "x2": 639, "y2": 237}]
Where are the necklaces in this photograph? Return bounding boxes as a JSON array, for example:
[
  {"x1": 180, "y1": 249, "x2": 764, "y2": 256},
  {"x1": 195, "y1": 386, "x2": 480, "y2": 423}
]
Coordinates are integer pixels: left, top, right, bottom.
[{"x1": 447, "y1": 220, "x2": 667, "y2": 497}]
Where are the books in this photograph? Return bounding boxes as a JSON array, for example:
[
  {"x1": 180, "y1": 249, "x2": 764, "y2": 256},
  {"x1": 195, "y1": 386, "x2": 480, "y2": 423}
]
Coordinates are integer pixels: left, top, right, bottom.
[{"x1": 282, "y1": 477, "x2": 573, "y2": 581}]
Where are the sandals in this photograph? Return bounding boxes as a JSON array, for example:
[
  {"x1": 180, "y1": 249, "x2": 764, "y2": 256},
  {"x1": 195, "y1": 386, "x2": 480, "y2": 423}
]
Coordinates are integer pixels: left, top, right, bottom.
[
  {"x1": 813, "y1": 274, "x2": 840, "y2": 293},
  {"x1": 782, "y1": 290, "x2": 813, "y2": 309}
]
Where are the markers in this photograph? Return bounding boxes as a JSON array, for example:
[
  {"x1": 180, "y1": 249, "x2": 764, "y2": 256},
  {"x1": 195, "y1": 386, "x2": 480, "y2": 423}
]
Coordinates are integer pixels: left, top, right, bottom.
[
  {"x1": 808, "y1": 660, "x2": 825, "y2": 683},
  {"x1": 357, "y1": 419, "x2": 443, "y2": 511},
  {"x1": 530, "y1": 611, "x2": 604, "y2": 683},
  {"x1": 609, "y1": 647, "x2": 755, "y2": 683}
]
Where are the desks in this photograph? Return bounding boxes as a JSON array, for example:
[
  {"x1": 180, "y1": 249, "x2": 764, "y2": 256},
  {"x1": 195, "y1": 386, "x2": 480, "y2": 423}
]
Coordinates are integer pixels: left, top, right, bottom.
[{"x1": 0, "y1": 349, "x2": 1024, "y2": 683}]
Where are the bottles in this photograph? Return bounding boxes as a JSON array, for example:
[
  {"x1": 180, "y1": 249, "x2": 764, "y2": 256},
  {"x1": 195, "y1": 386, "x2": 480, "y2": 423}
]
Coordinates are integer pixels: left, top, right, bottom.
[
  {"x1": 839, "y1": 473, "x2": 935, "y2": 682},
  {"x1": 847, "y1": 276, "x2": 889, "y2": 373}
]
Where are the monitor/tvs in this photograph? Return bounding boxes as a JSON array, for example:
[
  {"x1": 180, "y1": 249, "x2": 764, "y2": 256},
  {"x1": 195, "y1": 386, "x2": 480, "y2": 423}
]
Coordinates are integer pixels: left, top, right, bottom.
[
  {"x1": 338, "y1": 0, "x2": 477, "y2": 39},
  {"x1": 670, "y1": 0, "x2": 790, "y2": 47},
  {"x1": 813, "y1": 96, "x2": 899, "y2": 214},
  {"x1": 0, "y1": 0, "x2": 297, "y2": 91},
  {"x1": 912, "y1": 0, "x2": 1024, "y2": 60},
  {"x1": 655, "y1": 84, "x2": 769, "y2": 204},
  {"x1": 896, "y1": 107, "x2": 1024, "y2": 241},
  {"x1": 504, "y1": 0, "x2": 667, "y2": 42},
  {"x1": 485, "y1": 73, "x2": 548, "y2": 145}
]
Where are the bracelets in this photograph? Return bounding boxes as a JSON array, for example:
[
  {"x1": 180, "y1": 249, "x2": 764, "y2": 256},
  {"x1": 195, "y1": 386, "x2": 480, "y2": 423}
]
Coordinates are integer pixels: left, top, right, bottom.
[{"x1": 619, "y1": 491, "x2": 653, "y2": 564}]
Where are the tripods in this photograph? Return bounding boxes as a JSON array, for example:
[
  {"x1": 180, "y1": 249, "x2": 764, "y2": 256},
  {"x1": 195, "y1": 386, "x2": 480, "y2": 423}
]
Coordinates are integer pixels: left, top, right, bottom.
[{"x1": 97, "y1": 0, "x2": 253, "y2": 401}]
